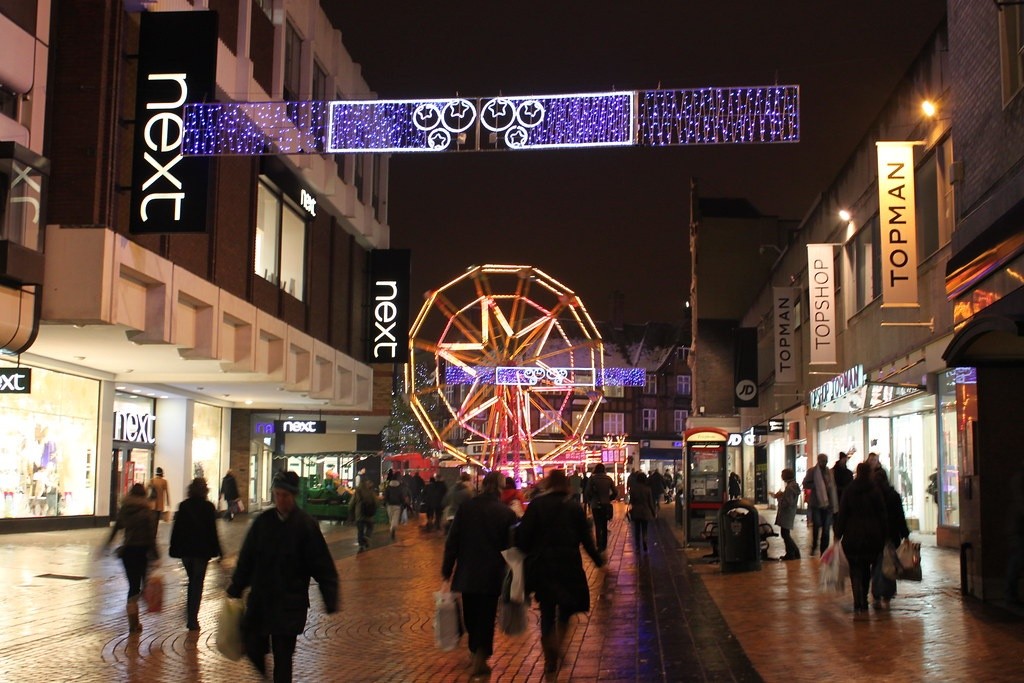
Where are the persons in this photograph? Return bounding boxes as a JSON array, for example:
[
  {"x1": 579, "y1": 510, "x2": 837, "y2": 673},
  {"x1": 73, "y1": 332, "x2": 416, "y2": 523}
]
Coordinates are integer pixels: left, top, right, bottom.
[
  {"x1": 626, "y1": 467, "x2": 684, "y2": 553},
  {"x1": 801, "y1": 453, "x2": 838, "y2": 557},
  {"x1": 169, "y1": 477, "x2": 220, "y2": 631},
  {"x1": 96, "y1": 483, "x2": 163, "y2": 633},
  {"x1": 580, "y1": 472, "x2": 591, "y2": 503},
  {"x1": 501, "y1": 476, "x2": 528, "y2": 634},
  {"x1": 518, "y1": 469, "x2": 608, "y2": 683},
  {"x1": 219, "y1": 470, "x2": 240, "y2": 521},
  {"x1": 347, "y1": 475, "x2": 378, "y2": 551},
  {"x1": 832, "y1": 446, "x2": 912, "y2": 621},
  {"x1": 728, "y1": 472, "x2": 742, "y2": 500},
  {"x1": 440, "y1": 471, "x2": 520, "y2": 677},
  {"x1": 227, "y1": 469, "x2": 340, "y2": 683},
  {"x1": 355, "y1": 467, "x2": 366, "y2": 488},
  {"x1": 774, "y1": 468, "x2": 801, "y2": 561},
  {"x1": 584, "y1": 463, "x2": 618, "y2": 552},
  {"x1": 382, "y1": 469, "x2": 447, "y2": 541},
  {"x1": 568, "y1": 470, "x2": 582, "y2": 505},
  {"x1": 146, "y1": 465, "x2": 170, "y2": 538}
]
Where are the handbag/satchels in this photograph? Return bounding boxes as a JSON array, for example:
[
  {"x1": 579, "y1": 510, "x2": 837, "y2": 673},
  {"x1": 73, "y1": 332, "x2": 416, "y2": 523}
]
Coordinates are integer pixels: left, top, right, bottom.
[
  {"x1": 146, "y1": 570, "x2": 164, "y2": 612},
  {"x1": 163, "y1": 508, "x2": 171, "y2": 521},
  {"x1": 432, "y1": 580, "x2": 465, "y2": 653},
  {"x1": 819, "y1": 539, "x2": 850, "y2": 593},
  {"x1": 214, "y1": 594, "x2": 246, "y2": 662},
  {"x1": 497, "y1": 562, "x2": 528, "y2": 636},
  {"x1": 236, "y1": 499, "x2": 244, "y2": 512},
  {"x1": 895, "y1": 539, "x2": 922, "y2": 581},
  {"x1": 114, "y1": 546, "x2": 127, "y2": 558},
  {"x1": 599, "y1": 502, "x2": 614, "y2": 520},
  {"x1": 882, "y1": 541, "x2": 904, "y2": 580}
]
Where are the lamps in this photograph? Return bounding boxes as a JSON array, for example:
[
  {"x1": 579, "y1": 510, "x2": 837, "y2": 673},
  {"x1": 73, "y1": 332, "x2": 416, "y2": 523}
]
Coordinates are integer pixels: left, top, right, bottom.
[{"x1": 838, "y1": 209, "x2": 853, "y2": 221}]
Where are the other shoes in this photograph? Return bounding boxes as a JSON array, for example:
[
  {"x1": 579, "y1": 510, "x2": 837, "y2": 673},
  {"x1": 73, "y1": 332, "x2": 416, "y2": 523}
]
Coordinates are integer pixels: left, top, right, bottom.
[
  {"x1": 186, "y1": 621, "x2": 200, "y2": 630},
  {"x1": 780, "y1": 556, "x2": 793, "y2": 560}
]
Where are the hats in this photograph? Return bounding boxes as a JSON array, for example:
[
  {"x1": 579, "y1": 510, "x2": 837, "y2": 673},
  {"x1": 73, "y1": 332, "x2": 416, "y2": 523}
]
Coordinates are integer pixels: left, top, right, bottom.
[
  {"x1": 840, "y1": 452, "x2": 847, "y2": 459},
  {"x1": 273, "y1": 471, "x2": 299, "y2": 492},
  {"x1": 156, "y1": 466, "x2": 163, "y2": 475}
]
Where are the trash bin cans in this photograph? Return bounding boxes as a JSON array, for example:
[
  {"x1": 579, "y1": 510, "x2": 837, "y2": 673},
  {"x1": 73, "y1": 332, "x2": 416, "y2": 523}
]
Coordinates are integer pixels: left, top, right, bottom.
[{"x1": 717, "y1": 499, "x2": 762, "y2": 574}]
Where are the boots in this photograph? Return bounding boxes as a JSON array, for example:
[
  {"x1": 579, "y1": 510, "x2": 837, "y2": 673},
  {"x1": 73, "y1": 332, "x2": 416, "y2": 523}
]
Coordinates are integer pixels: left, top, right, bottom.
[{"x1": 126, "y1": 604, "x2": 143, "y2": 632}]
